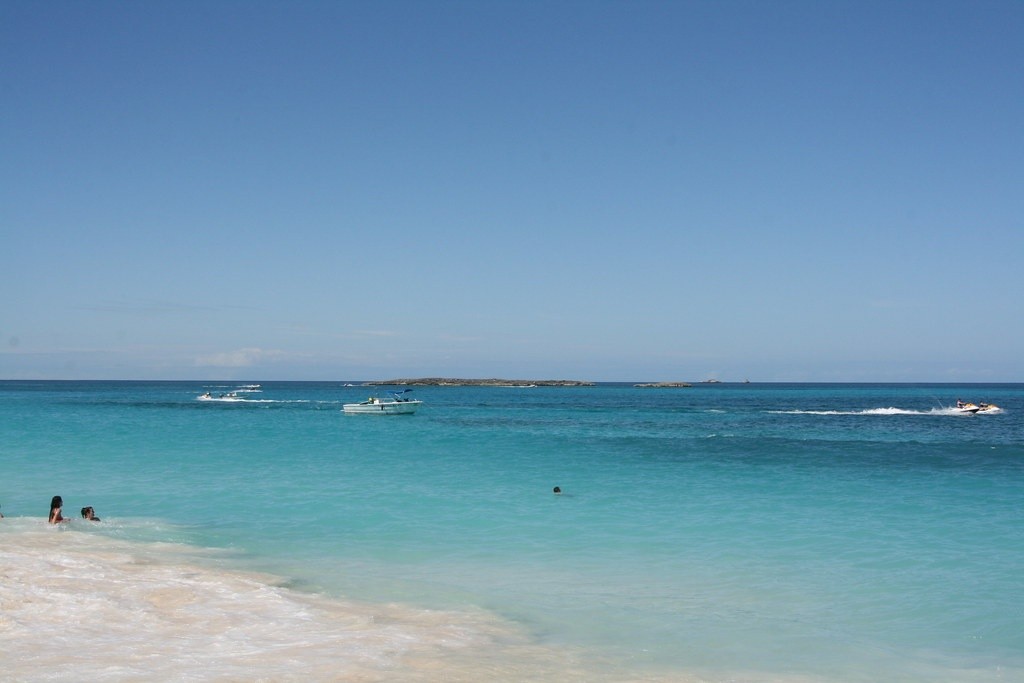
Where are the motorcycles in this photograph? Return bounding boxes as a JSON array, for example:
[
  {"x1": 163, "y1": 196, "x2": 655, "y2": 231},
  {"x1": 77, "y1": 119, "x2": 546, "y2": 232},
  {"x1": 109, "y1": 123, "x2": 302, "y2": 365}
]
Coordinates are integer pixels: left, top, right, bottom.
[
  {"x1": 970, "y1": 403, "x2": 1003, "y2": 416},
  {"x1": 951, "y1": 402, "x2": 980, "y2": 413}
]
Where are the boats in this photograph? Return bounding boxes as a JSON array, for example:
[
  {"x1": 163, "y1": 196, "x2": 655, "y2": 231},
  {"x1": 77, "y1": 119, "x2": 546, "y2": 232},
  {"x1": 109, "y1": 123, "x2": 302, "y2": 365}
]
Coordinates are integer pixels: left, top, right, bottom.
[{"x1": 342, "y1": 388, "x2": 425, "y2": 415}]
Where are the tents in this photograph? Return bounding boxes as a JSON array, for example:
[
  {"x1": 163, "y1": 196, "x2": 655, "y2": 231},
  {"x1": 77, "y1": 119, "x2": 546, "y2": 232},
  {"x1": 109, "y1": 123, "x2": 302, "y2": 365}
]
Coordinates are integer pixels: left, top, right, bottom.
[{"x1": 388, "y1": 388, "x2": 413, "y2": 394}]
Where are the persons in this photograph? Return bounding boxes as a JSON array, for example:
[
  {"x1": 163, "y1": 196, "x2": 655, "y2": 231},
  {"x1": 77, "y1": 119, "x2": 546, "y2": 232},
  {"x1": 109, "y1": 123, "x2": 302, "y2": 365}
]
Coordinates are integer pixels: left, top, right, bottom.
[
  {"x1": 81, "y1": 506, "x2": 99, "y2": 522},
  {"x1": 957, "y1": 399, "x2": 966, "y2": 408},
  {"x1": 554, "y1": 487, "x2": 560, "y2": 492},
  {"x1": 49, "y1": 496, "x2": 64, "y2": 523},
  {"x1": 404, "y1": 398, "x2": 409, "y2": 402},
  {"x1": 980, "y1": 401, "x2": 983, "y2": 407}
]
